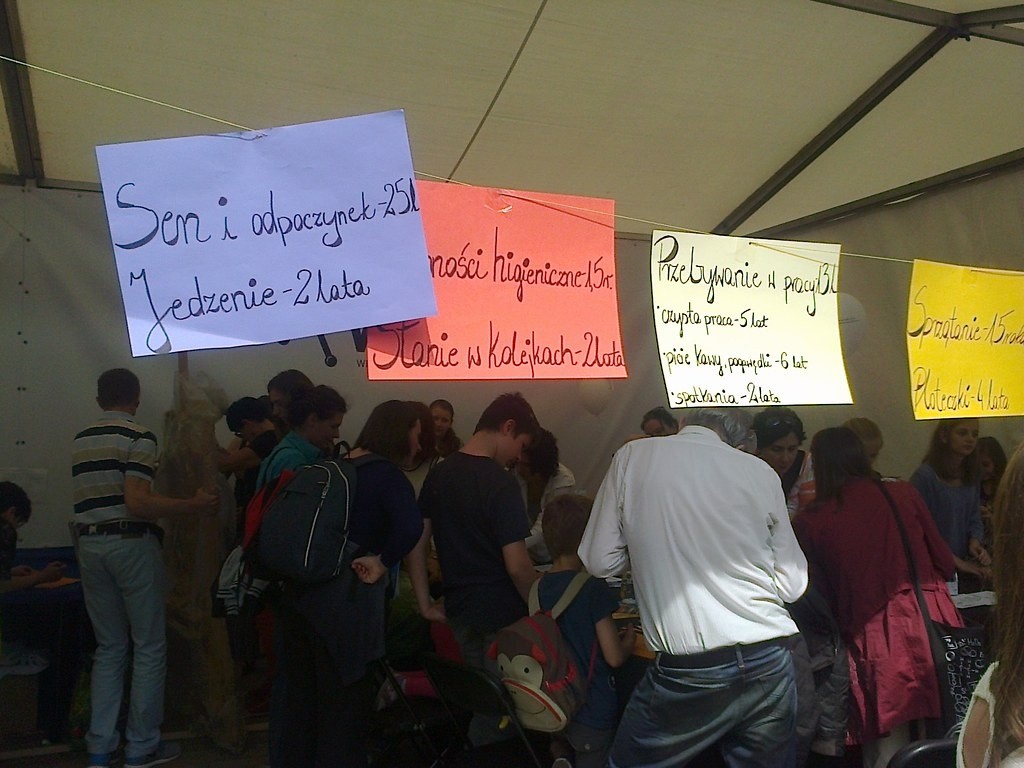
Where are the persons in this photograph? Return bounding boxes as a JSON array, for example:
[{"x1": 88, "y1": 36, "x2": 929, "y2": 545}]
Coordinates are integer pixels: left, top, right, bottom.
[
  {"x1": 956, "y1": 441, "x2": 1024, "y2": 768},
  {"x1": 0, "y1": 369, "x2": 1008, "y2": 768}
]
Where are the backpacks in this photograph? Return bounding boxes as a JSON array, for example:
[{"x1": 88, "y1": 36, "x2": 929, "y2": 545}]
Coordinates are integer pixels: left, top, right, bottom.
[
  {"x1": 495, "y1": 569, "x2": 597, "y2": 732},
  {"x1": 262, "y1": 439, "x2": 380, "y2": 582}
]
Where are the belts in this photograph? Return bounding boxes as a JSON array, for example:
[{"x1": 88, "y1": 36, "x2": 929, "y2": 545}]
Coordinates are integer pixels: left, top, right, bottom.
[
  {"x1": 79, "y1": 523, "x2": 156, "y2": 537},
  {"x1": 656, "y1": 636, "x2": 778, "y2": 669}
]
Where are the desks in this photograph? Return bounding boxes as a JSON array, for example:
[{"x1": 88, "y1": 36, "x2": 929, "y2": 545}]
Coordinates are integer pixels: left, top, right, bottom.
[{"x1": 0, "y1": 546, "x2": 86, "y2": 741}]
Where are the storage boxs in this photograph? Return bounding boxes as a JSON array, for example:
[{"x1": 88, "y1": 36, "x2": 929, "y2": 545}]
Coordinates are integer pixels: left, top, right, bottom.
[{"x1": 1, "y1": 674, "x2": 45, "y2": 737}]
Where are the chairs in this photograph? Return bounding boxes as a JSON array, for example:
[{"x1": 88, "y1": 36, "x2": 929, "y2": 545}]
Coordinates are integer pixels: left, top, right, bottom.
[{"x1": 367, "y1": 652, "x2": 547, "y2": 768}]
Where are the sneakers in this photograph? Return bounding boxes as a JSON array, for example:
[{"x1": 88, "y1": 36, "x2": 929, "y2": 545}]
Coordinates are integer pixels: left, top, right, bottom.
[
  {"x1": 125, "y1": 741, "x2": 182, "y2": 768},
  {"x1": 88, "y1": 742, "x2": 125, "y2": 768}
]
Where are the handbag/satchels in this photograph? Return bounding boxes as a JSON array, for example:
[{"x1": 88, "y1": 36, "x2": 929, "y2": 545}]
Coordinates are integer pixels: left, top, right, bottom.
[{"x1": 927, "y1": 616, "x2": 989, "y2": 730}]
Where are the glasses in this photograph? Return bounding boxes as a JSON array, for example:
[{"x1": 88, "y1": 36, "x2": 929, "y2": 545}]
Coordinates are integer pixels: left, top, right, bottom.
[
  {"x1": 765, "y1": 416, "x2": 795, "y2": 428},
  {"x1": 235, "y1": 420, "x2": 244, "y2": 439}
]
